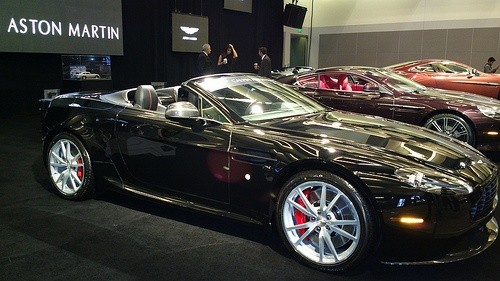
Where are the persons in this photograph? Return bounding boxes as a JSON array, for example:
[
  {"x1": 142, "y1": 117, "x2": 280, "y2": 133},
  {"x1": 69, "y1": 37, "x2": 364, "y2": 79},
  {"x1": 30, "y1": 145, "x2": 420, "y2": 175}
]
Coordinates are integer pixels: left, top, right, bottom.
[
  {"x1": 217, "y1": 44, "x2": 238, "y2": 73},
  {"x1": 197, "y1": 44, "x2": 213, "y2": 75},
  {"x1": 484, "y1": 57, "x2": 498, "y2": 74},
  {"x1": 255, "y1": 47, "x2": 272, "y2": 78}
]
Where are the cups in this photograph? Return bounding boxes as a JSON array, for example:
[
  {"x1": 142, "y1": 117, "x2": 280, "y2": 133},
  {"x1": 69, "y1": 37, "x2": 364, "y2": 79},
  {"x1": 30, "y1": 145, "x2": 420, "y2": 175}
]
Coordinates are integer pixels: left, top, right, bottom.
[
  {"x1": 224, "y1": 58, "x2": 227, "y2": 64},
  {"x1": 254, "y1": 63, "x2": 258, "y2": 69}
]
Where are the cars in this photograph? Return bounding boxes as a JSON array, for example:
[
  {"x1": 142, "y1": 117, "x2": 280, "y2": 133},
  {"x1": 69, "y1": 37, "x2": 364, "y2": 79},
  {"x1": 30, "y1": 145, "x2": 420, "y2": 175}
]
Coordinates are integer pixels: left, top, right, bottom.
[{"x1": 75, "y1": 72, "x2": 101, "y2": 79}]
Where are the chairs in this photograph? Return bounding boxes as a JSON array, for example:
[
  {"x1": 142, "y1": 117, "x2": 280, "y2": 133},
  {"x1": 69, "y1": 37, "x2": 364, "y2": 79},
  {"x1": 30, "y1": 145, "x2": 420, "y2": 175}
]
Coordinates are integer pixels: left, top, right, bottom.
[
  {"x1": 337, "y1": 75, "x2": 352, "y2": 91},
  {"x1": 320, "y1": 76, "x2": 330, "y2": 89},
  {"x1": 134, "y1": 85, "x2": 167, "y2": 112},
  {"x1": 319, "y1": 80, "x2": 326, "y2": 88},
  {"x1": 202, "y1": 106, "x2": 228, "y2": 123}
]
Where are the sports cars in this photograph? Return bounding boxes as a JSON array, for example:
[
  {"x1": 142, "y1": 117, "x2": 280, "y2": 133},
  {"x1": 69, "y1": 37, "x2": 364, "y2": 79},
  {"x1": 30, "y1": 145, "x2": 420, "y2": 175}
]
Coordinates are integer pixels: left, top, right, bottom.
[
  {"x1": 273, "y1": 66, "x2": 500, "y2": 160},
  {"x1": 382, "y1": 58, "x2": 500, "y2": 101},
  {"x1": 269, "y1": 66, "x2": 315, "y2": 81},
  {"x1": 40, "y1": 71, "x2": 500, "y2": 274}
]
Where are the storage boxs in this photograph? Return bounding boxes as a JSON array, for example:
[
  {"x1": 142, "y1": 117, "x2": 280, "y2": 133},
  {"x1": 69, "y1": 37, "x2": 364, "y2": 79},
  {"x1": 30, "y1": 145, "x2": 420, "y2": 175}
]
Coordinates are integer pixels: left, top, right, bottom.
[{"x1": 60, "y1": 54, "x2": 111, "y2": 80}]
